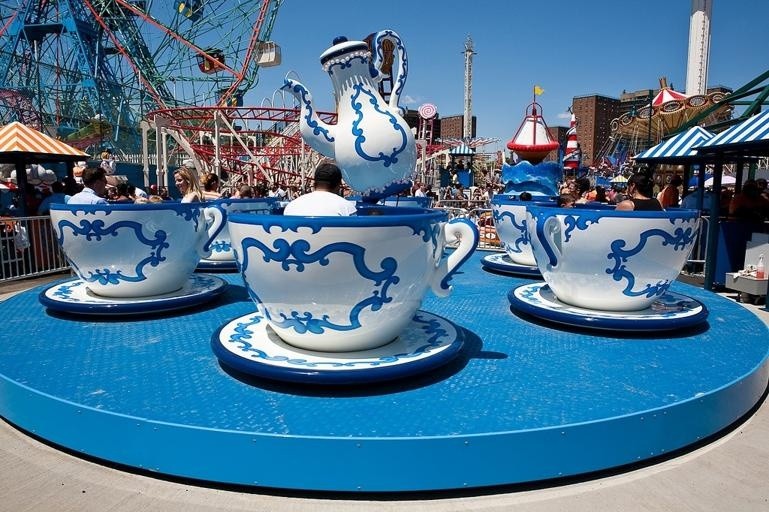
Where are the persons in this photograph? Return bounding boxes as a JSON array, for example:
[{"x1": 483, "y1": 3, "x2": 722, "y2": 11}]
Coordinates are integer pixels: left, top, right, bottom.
[
  {"x1": 733, "y1": 181, "x2": 766, "y2": 268},
  {"x1": 173, "y1": 166, "x2": 205, "y2": 204},
  {"x1": 414, "y1": 176, "x2": 500, "y2": 229},
  {"x1": 198, "y1": 173, "x2": 222, "y2": 200},
  {"x1": 338, "y1": 183, "x2": 352, "y2": 199},
  {"x1": 38, "y1": 182, "x2": 71, "y2": 215},
  {"x1": 67, "y1": 164, "x2": 110, "y2": 205},
  {"x1": 558, "y1": 176, "x2": 769, "y2": 217},
  {"x1": 282, "y1": 163, "x2": 356, "y2": 217},
  {"x1": 230, "y1": 181, "x2": 314, "y2": 200},
  {"x1": 105, "y1": 183, "x2": 171, "y2": 204}
]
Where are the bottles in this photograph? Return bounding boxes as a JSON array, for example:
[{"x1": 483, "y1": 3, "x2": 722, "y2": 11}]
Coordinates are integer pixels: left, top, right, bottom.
[{"x1": 757, "y1": 257, "x2": 764, "y2": 279}]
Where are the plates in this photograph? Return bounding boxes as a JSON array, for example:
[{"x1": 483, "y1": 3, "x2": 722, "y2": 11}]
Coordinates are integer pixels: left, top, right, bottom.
[
  {"x1": 506, "y1": 284, "x2": 706, "y2": 332},
  {"x1": 212, "y1": 306, "x2": 469, "y2": 386},
  {"x1": 480, "y1": 250, "x2": 540, "y2": 276},
  {"x1": 40, "y1": 278, "x2": 228, "y2": 318}
]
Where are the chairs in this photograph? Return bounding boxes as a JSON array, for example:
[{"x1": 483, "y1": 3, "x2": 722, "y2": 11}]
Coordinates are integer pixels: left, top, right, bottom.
[{"x1": 725, "y1": 240, "x2": 769, "y2": 301}]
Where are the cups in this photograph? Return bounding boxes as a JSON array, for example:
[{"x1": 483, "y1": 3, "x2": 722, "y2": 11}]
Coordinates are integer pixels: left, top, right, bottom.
[
  {"x1": 490, "y1": 192, "x2": 619, "y2": 264},
  {"x1": 190, "y1": 198, "x2": 278, "y2": 263},
  {"x1": 49, "y1": 203, "x2": 227, "y2": 300},
  {"x1": 224, "y1": 208, "x2": 478, "y2": 353},
  {"x1": 524, "y1": 202, "x2": 706, "y2": 312}
]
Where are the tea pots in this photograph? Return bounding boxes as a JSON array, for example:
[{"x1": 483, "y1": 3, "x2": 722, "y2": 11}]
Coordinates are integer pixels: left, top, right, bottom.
[{"x1": 279, "y1": 29, "x2": 418, "y2": 200}]
